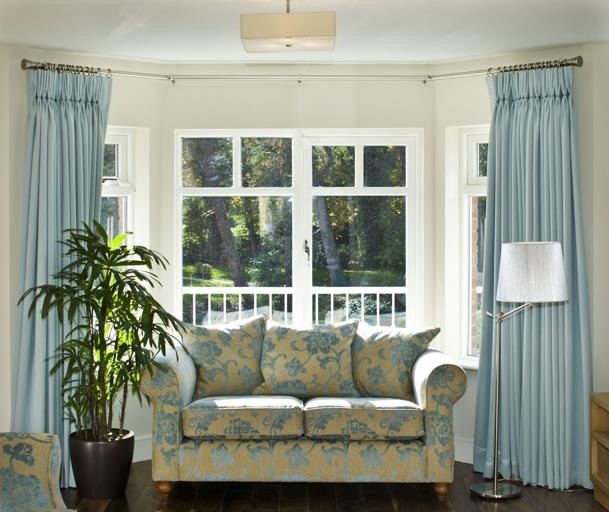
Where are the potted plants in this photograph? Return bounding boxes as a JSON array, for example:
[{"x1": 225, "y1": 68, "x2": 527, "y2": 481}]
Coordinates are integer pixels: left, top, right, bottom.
[{"x1": 18, "y1": 219, "x2": 192, "y2": 500}]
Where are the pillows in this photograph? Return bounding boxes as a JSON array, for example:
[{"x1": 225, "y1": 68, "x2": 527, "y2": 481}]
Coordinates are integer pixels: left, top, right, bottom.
[
  {"x1": 351, "y1": 322, "x2": 441, "y2": 403},
  {"x1": 176, "y1": 313, "x2": 268, "y2": 403},
  {"x1": 252, "y1": 317, "x2": 359, "y2": 397}
]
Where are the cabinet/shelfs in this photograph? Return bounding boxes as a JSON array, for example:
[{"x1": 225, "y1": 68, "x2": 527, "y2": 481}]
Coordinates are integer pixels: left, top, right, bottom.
[{"x1": 590, "y1": 392, "x2": 609, "y2": 511}]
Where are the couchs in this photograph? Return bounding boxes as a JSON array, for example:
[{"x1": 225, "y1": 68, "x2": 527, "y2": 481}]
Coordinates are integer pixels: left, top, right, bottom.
[
  {"x1": 0, "y1": 432, "x2": 77, "y2": 512},
  {"x1": 141, "y1": 333, "x2": 468, "y2": 502}
]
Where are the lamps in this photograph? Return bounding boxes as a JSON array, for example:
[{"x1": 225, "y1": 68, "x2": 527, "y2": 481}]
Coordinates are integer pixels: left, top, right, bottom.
[
  {"x1": 240, "y1": 0, "x2": 336, "y2": 53},
  {"x1": 469, "y1": 241, "x2": 569, "y2": 502}
]
